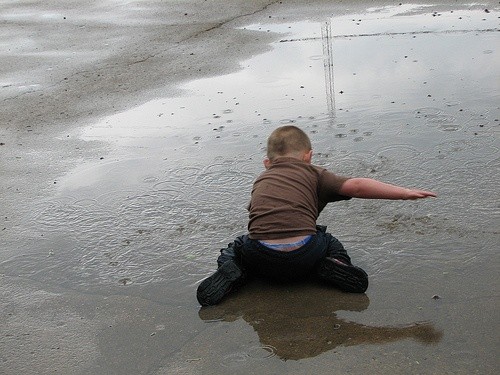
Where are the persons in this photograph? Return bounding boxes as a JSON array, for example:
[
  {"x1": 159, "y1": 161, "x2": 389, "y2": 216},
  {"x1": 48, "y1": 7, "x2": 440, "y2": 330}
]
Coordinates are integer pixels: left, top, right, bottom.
[{"x1": 195, "y1": 126, "x2": 436, "y2": 306}]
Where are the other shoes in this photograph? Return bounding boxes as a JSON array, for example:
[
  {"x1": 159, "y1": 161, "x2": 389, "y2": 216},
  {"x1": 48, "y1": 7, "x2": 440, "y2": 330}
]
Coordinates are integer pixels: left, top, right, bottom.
[
  {"x1": 196, "y1": 259, "x2": 245, "y2": 307},
  {"x1": 318, "y1": 257, "x2": 369, "y2": 293}
]
